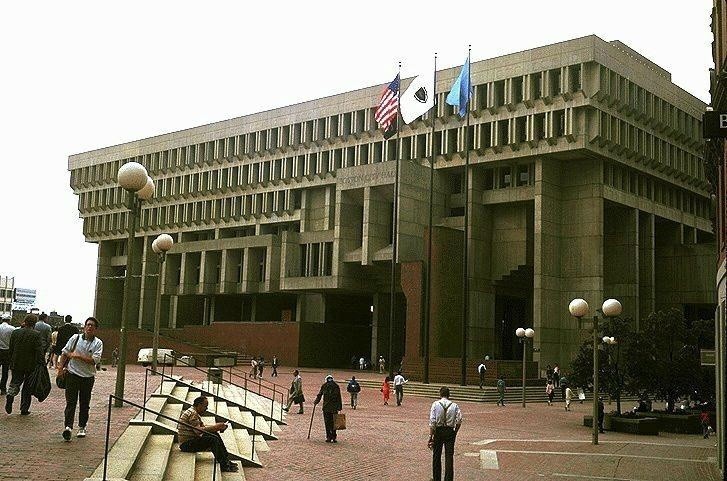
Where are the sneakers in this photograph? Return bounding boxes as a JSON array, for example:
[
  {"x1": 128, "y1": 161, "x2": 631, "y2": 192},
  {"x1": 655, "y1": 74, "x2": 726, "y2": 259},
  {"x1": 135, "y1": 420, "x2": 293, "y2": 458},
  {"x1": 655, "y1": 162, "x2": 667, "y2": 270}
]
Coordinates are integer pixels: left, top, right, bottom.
[
  {"x1": 61, "y1": 426, "x2": 73, "y2": 442},
  {"x1": 222, "y1": 461, "x2": 240, "y2": 473},
  {"x1": 77, "y1": 427, "x2": 87, "y2": 438},
  {"x1": 325, "y1": 440, "x2": 337, "y2": 443},
  {"x1": 282, "y1": 408, "x2": 303, "y2": 414},
  {"x1": 4, "y1": 394, "x2": 14, "y2": 415},
  {"x1": 21, "y1": 410, "x2": 31, "y2": 415}
]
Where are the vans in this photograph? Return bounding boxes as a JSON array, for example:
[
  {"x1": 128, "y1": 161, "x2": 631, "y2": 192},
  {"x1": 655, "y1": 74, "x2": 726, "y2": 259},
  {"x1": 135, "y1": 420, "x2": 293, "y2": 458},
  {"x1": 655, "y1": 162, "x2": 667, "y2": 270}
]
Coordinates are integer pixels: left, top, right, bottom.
[{"x1": 135, "y1": 347, "x2": 176, "y2": 367}]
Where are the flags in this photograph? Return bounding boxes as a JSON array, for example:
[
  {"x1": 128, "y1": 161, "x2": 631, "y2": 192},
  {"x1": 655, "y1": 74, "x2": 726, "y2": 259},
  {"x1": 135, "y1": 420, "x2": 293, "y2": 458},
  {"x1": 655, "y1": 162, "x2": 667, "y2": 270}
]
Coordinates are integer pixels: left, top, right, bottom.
[
  {"x1": 373, "y1": 71, "x2": 399, "y2": 141},
  {"x1": 400, "y1": 60, "x2": 436, "y2": 125},
  {"x1": 446, "y1": 55, "x2": 472, "y2": 118}
]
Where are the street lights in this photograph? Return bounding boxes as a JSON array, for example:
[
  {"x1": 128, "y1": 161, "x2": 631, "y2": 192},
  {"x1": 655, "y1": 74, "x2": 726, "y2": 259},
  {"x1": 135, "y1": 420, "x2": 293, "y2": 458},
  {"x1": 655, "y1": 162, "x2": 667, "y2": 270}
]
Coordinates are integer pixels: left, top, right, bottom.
[
  {"x1": 515, "y1": 327, "x2": 534, "y2": 408},
  {"x1": 602, "y1": 335, "x2": 619, "y2": 406},
  {"x1": 568, "y1": 296, "x2": 624, "y2": 446},
  {"x1": 113, "y1": 161, "x2": 158, "y2": 409},
  {"x1": 150, "y1": 232, "x2": 175, "y2": 374}
]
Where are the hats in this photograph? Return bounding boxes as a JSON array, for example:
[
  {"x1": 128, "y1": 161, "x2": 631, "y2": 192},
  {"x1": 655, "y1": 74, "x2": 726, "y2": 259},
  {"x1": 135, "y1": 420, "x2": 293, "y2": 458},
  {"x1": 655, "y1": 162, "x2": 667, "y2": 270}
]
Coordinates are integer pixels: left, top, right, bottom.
[
  {"x1": 1, "y1": 313, "x2": 13, "y2": 321},
  {"x1": 23, "y1": 313, "x2": 38, "y2": 326}
]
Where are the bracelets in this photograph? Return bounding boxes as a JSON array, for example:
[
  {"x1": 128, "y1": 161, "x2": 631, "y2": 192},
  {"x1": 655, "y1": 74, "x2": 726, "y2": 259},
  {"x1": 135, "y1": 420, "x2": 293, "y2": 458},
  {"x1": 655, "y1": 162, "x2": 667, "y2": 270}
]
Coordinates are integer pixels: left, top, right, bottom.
[{"x1": 428, "y1": 434, "x2": 434, "y2": 438}]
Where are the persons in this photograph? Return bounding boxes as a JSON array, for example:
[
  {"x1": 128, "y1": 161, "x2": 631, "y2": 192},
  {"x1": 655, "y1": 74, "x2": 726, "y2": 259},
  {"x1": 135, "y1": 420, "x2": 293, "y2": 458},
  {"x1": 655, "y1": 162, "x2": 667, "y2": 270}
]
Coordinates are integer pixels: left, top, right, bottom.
[
  {"x1": 428, "y1": 386, "x2": 463, "y2": 481},
  {"x1": 680, "y1": 400, "x2": 698, "y2": 410},
  {"x1": 392, "y1": 371, "x2": 410, "y2": 408},
  {"x1": 312, "y1": 371, "x2": 341, "y2": 442},
  {"x1": 359, "y1": 356, "x2": 364, "y2": 369},
  {"x1": 637, "y1": 399, "x2": 647, "y2": 410},
  {"x1": 250, "y1": 353, "x2": 279, "y2": 379},
  {"x1": 282, "y1": 369, "x2": 306, "y2": 414},
  {"x1": 701, "y1": 409, "x2": 710, "y2": 441},
  {"x1": 477, "y1": 362, "x2": 487, "y2": 391},
  {"x1": 0, "y1": 311, "x2": 103, "y2": 441},
  {"x1": 178, "y1": 395, "x2": 239, "y2": 474},
  {"x1": 546, "y1": 361, "x2": 586, "y2": 412},
  {"x1": 347, "y1": 376, "x2": 360, "y2": 411},
  {"x1": 496, "y1": 377, "x2": 506, "y2": 407},
  {"x1": 378, "y1": 355, "x2": 385, "y2": 373},
  {"x1": 382, "y1": 376, "x2": 392, "y2": 406},
  {"x1": 598, "y1": 397, "x2": 604, "y2": 435}
]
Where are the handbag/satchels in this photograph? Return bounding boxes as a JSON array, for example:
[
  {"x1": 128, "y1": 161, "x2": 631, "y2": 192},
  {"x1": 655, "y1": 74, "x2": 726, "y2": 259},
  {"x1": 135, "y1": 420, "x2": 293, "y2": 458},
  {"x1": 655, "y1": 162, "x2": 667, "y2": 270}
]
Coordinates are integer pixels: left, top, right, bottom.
[
  {"x1": 294, "y1": 394, "x2": 305, "y2": 404},
  {"x1": 56, "y1": 368, "x2": 68, "y2": 389},
  {"x1": 332, "y1": 412, "x2": 347, "y2": 430},
  {"x1": 26, "y1": 363, "x2": 51, "y2": 403}
]
